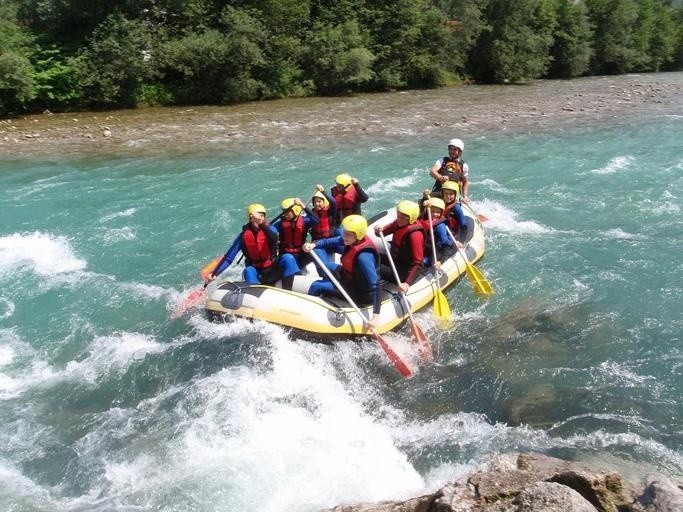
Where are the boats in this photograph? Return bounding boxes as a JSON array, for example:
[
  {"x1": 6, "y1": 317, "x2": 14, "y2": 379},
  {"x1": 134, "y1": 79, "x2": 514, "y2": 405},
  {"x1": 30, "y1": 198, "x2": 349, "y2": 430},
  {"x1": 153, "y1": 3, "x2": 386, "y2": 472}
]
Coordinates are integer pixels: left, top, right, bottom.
[{"x1": 205, "y1": 201, "x2": 487, "y2": 345}]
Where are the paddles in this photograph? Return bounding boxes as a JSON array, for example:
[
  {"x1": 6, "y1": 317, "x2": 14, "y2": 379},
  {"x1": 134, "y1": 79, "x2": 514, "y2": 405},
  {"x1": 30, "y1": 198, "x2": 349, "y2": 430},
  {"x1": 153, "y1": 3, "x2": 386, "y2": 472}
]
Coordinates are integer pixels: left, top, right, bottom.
[
  {"x1": 423, "y1": 188, "x2": 492, "y2": 296},
  {"x1": 372, "y1": 221, "x2": 437, "y2": 365},
  {"x1": 419, "y1": 198, "x2": 450, "y2": 320},
  {"x1": 171, "y1": 213, "x2": 254, "y2": 317},
  {"x1": 442, "y1": 177, "x2": 488, "y2": 223},
  {"x1": 306, "y1": 243, "x2": 413, "y2": 380}
]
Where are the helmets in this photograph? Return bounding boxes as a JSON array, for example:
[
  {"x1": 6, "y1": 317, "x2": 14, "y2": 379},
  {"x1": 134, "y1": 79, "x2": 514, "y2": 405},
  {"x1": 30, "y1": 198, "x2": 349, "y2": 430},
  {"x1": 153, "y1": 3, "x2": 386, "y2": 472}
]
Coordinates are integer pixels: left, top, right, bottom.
[
  {"x1": 423, "y1": 197, "x2": 446, "y2": 218},
  {"x1": 247, "y1": 203, "x2": 266, "y2": 219},
  {"x1": 446, "y1": 138, "x2": 463, "y2": 152},
  {"x1": 280, "y1": 197, "x2": 302, "y2": 216},
  {"x1": 340, "y1": 214, "x2": 368, "y2": 241},
  {"x1": 311, "y1": 191, "x2": 328, "y2": 206},
  {"x1": 396, "y1": 200, "x2": 420, "y2": 224},
  {"x1": 440, "y1": 181, "x2": 459, "y2": 197},
  {"x1": 334, "y1": 173, "x2": 352, "y2": 191}
]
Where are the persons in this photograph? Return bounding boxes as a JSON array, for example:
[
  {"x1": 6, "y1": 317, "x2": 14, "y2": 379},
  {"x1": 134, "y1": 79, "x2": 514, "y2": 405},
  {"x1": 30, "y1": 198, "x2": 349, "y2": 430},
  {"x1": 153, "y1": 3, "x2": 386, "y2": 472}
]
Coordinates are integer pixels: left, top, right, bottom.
[
  {"x1": 423, "y1": 181, "x2": 467, "y2": 248},
  {"x1": 331, "y1": 172, "x2": 369, "y2": 227},
  {"x1": 302, "y1": 215, "x2": 381, "y2": 333},
  {"x1": 306, "y1": 184, "x2": 337, "y2": 241},
  {"x1": 274, "y1": 198, "x2": 329, "y2": 277},
  {"x1": 373, "y1": 200, "x2": 426, "y2": 294},
  {"x1": 417, "y1": 197, "x2": 448, "y2": 270},
  {"x1": 429, "y1": 138, "x2": 468, "y2": 204},
  {"x1": 207, "y1": 203, "x2": 301, "y2": 290}
]
